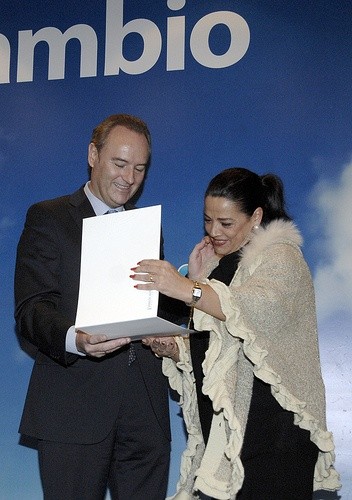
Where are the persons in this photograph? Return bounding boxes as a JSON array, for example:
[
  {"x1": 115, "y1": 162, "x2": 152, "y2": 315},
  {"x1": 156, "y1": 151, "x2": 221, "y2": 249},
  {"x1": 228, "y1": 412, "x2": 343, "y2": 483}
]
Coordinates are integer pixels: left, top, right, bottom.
[
  {"x1": 14, "y1": 114, "x2": 172, "y2": 500},
  {"x1": 127, "y1": 167, "x2": 342, "y2": 499}
]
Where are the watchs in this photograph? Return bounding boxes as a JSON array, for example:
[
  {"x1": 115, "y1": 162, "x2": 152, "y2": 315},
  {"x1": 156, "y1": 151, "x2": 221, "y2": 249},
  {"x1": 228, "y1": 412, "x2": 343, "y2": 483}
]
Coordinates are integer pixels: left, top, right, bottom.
[{"x1": 186, "y1": 280, "x2": 202, "y2": 308}]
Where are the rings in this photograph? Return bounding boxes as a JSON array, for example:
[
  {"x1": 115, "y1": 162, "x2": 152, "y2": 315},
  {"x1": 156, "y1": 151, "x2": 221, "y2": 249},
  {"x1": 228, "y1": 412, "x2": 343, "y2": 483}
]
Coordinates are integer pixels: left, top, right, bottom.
[{"x1": 150, "y1": 275, "x2": 152, "y2": 281}]
